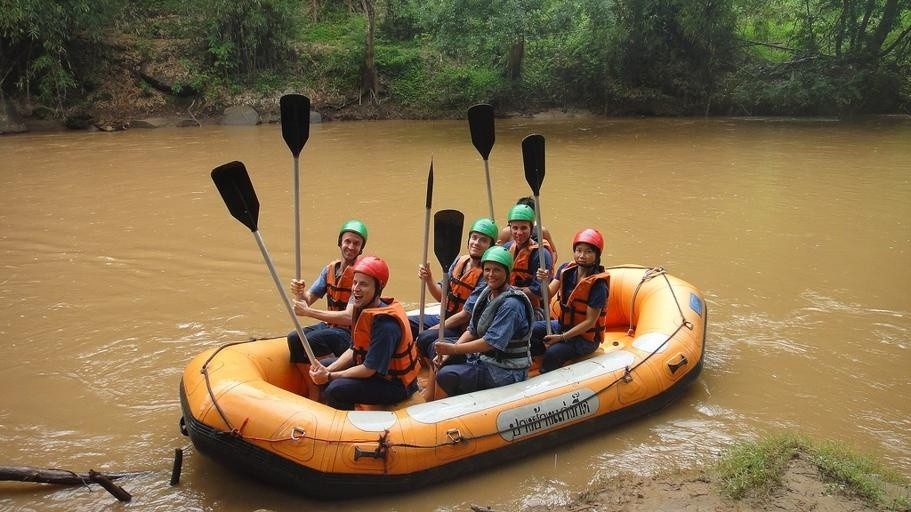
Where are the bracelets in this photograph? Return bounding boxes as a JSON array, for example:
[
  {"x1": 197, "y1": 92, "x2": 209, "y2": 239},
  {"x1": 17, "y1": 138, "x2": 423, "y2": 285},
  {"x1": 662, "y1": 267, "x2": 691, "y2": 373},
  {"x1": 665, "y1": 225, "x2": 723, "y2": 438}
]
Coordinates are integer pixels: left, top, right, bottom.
[{"x1": 327, "y1": 372, "x2": 332, "y2": 382}]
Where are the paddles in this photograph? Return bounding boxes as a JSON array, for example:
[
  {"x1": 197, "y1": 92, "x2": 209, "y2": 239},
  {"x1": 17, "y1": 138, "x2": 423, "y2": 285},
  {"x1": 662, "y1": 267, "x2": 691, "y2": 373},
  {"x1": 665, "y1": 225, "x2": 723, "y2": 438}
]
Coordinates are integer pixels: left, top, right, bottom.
[
  {"x1": 467, "y1": 103, "x2": 495, "y2": 222},
  {"x1": 279, "y1": 94, "x2": 310, "y2": 302},
  {"x1": 433, "y1": 209, "x2": 465, "y2": 371},
  {"x1": 521, "y1": 134, "x2": 552, "y2": 337},
  {"x1": 211, "y1": 160, "x2": 319, "y2": 374},
  {"x1": 418, "y1": 152, "x2": 434, "y2": 335}
]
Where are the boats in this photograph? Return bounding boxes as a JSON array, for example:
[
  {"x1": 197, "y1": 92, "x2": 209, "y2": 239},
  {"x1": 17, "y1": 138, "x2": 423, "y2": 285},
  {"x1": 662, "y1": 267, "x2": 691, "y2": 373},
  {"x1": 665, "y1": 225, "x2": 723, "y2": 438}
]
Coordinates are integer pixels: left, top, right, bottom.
[{"x1": 179, "y1": 264, "x2": 707, "y2": 503}]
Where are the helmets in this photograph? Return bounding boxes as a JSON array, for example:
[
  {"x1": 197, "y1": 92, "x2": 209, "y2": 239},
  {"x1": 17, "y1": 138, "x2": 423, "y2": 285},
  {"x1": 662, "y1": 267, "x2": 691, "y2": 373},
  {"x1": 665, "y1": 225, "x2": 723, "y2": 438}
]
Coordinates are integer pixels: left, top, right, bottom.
[
  {"x1": 469, "y1": 218, "x2": 498, "y2": 246},
  {"x1": 337, "y1": 220, "x2": 368, "y2": 248},
  {"x1": 352, "y1": 256, "x2": 389, "y2": 289},
  {"x1": 573, "y1": 228, "x2": 603, "y2": 254},
  {"x1": 481, "y1": 246, "x2": 513, "y2": 275},
  {"x1": 508, "y1": 204, "x2": 535, "y2": 225}
]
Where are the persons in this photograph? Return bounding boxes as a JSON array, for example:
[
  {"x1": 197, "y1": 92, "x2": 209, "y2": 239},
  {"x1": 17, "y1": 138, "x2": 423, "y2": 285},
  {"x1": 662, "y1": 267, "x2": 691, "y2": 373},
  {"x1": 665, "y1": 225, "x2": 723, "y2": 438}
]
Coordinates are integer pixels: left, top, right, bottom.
[
  {"x1": 495, "y1": 205, "x2": 551, "y2": 321},
  {"x1": 310, "y1": 256, "x2": 422, "y2": 412},
  {"x1": 434, "y1": 245, "x2": 535, "y2": 395},
  {"x1": 530, "y1": 229, "x2": 610, "y2": 374},
  {"x1": 288, "y1": 218, "x2": 367, "y2": 404},
  {"x1": 499, "y1": 198, "x2": 557, "y2": 283},
  {"x1": 406, "y1": 218, "x2": 499, "y2": 370}
]
